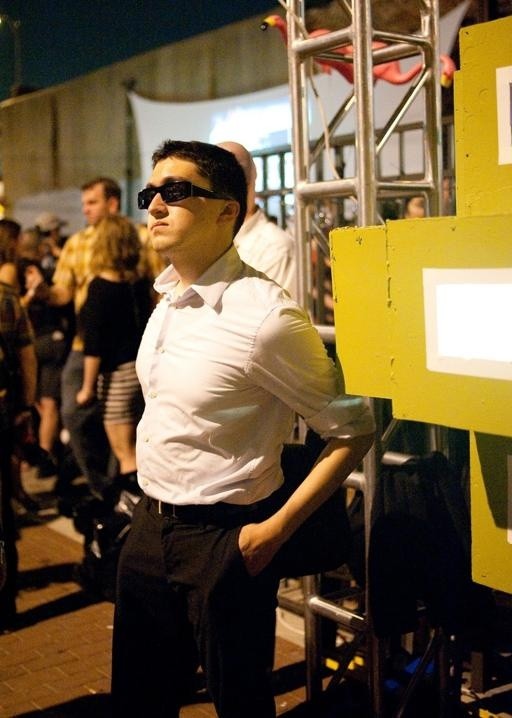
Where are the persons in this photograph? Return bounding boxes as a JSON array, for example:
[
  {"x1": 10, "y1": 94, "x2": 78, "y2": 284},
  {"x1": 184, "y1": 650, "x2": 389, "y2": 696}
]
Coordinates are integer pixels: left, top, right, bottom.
[
  {"x1": 111, "y1": 139, "x2": 378, "y2": 718},
  {"x1": 1, "y1": 142, "x2": 456, "y2": 634}
]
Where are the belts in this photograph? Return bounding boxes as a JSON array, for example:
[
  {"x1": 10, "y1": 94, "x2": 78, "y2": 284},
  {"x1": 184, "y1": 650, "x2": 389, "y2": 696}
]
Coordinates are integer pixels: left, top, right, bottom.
[{"x1": 144, "y1": 494, "x2": 250, "y2": 521}]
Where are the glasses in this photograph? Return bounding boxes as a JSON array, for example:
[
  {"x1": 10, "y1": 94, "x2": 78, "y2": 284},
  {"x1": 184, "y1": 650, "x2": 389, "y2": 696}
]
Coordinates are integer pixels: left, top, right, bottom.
[{"x1": 138, "y1": 180, "x2": 222, "y2": 208}]
